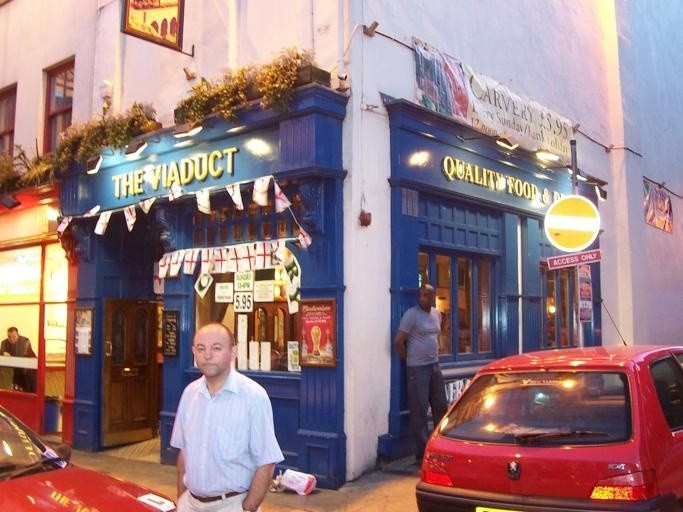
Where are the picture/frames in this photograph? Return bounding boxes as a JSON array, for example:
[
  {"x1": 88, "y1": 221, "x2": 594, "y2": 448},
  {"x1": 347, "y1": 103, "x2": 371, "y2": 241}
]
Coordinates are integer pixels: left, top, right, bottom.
[
  {"x1": 72, "y1": 307, "x2": 93, "y2": 357},
  {"x1": 297, "y1": 298, "x2": 337, "y2": 368},
  {"x1": 161, "y1": 309, "x2": 179, "y2": 357}
]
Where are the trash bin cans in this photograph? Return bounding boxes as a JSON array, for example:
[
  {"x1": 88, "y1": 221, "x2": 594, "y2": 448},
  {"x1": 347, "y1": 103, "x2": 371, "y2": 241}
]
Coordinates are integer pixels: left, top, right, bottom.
[{"x1": 44, "y1": 395, "x2": 59, "y2": 432}]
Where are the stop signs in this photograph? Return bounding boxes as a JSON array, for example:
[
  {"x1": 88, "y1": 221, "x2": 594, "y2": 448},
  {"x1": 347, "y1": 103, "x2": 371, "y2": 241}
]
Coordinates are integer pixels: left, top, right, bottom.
[{"x1": 543, "y1": 194, "x2": 602, "y2": 254}]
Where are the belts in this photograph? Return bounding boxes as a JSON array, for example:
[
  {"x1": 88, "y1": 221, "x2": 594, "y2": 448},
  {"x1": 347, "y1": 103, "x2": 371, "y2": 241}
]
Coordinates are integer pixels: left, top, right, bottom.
[{"x1": 188, "y1": 488, "x2": 248, "y2": 504}]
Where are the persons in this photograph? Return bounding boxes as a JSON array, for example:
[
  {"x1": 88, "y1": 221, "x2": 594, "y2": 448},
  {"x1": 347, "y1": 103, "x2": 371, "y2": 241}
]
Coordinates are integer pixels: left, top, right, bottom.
[
  {"x1": 394, "y1": 282, "x2": 449, "y2": 471},
  {"x1": 170, "y1": 322, "x2": 284, "y2": 512},
  {"x1": 1, "y1": 326, "x2": 39, "y2": 393}
]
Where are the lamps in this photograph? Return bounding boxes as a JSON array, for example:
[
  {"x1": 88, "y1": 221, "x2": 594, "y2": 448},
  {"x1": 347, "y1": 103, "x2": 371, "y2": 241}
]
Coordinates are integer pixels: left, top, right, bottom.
[
  {"x1": 171, "y1": 121, "x2": 202, "y2": 139},
  {"x1": 85, "y1": 148, "x2": 114, "y2": 175},
  {"x1": 124, "y1": 134, "x2": 161, "y2": 159},
  {"x1": 530, "y1": 148, "x2": 607, "y2": 201},
  {"x1": 456, "y1": 132, "x2": 519, "y2": 150}
]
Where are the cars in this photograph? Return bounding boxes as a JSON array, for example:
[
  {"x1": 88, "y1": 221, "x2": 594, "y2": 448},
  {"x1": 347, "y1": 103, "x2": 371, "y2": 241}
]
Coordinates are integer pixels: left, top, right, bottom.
[
  {"x1": 1, "y1": 403, "x2": 178, "y2": 511},
  {"x1": 414, "y1": 343, "x2": 682, "y2": 511}
]
